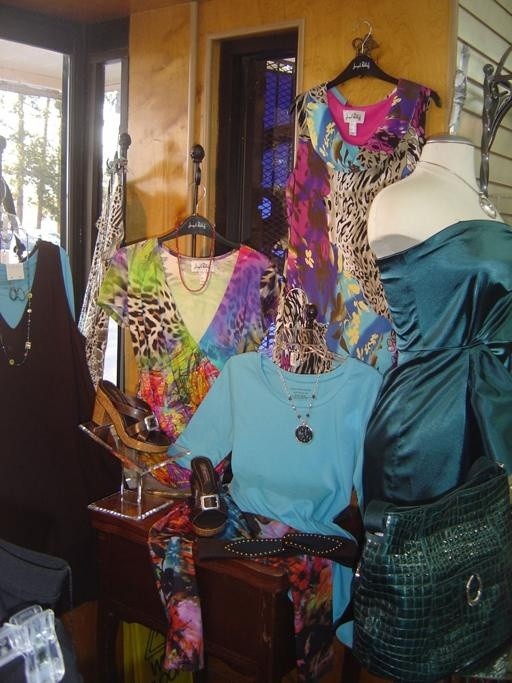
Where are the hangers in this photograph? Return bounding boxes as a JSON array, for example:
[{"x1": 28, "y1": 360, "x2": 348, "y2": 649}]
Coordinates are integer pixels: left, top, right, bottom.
[
  {"x1": 158, "y1": 181, "x2": 239, "y2": 250},
  {"x1": 288, "y1": 20, "x2": 440, "y2": 115},
  {"x1": 0, "y1": 178, "x2": 36, "y2": 243}
]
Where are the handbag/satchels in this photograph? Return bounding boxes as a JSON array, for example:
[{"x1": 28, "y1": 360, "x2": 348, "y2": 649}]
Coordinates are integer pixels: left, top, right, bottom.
[{"x1": 352, "y1": 461, "x2": 512, "y2": 683}]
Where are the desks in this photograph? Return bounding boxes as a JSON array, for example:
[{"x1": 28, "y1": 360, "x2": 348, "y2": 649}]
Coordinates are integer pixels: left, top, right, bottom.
[{"x1": 87, "y1": 488, "x2": 296, "y2": 683}]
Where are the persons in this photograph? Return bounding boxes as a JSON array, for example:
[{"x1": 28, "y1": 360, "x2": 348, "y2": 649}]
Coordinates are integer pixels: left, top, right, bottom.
[{"x1": 360, "y1": 137, "x2": 512, "y2": 506}]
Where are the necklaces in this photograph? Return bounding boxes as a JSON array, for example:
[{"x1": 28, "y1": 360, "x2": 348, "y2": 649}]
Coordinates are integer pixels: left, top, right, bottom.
[
  {"x1": 0, "y1": 233, "x2": 34, "y2": 369},
  {"x1": 414, "y1": 160, "x2": 497, "y2": 219},
  {"x1": 274, "y1": 361, "x2": 325, "y2": 443},
  {"x1": 174, "y1": 223, "x2": 216, "y2": 292}
]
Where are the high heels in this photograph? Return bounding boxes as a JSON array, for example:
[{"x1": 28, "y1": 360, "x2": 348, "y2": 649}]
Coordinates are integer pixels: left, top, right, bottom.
[
  {"x1": 92, "y1": 380, "x2": 170, "y2": 454},
  {"x1": 191, "y1": 456, "x2": 229, "y2": 538}
]
